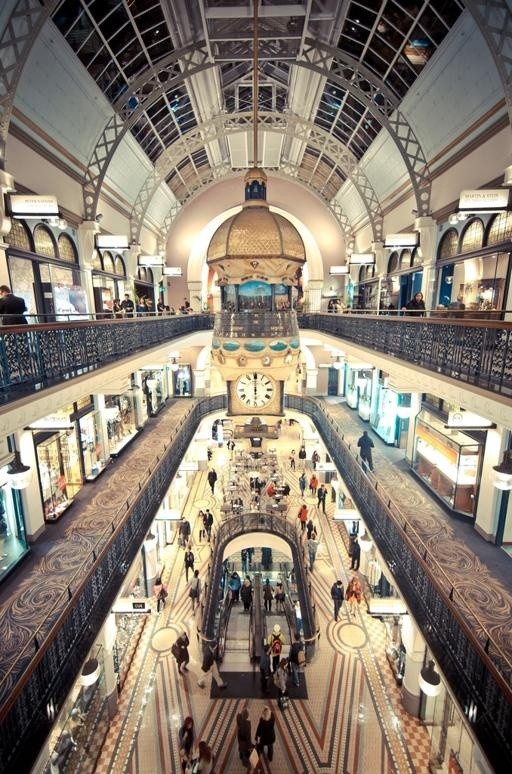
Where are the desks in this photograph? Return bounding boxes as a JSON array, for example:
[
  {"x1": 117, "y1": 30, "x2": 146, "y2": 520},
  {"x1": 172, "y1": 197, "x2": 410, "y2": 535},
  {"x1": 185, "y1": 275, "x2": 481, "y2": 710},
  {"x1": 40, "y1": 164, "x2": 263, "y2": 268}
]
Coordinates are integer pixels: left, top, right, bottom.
[{"x1": 222, "y1": 447, "x2": 288, "y2": 518}]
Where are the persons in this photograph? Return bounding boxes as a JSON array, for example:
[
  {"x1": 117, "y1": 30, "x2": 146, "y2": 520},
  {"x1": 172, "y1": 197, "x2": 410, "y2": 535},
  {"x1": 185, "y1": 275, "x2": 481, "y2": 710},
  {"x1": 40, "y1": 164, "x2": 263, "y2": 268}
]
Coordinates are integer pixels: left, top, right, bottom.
[
  {"x1": 1, "y1": 284, "x2": 30, "y2": 384},
  {"x1": 326, "y1": 292, "x2": 427, "y2": 316},
  {"x1": 357, "y1": 430, "x2": 375, "y2": 473},
  {"x1": 103, "y1": 293, "x2": 193, "y2": 316},
  {"x1": 153, "y1": 445, "x2": 360, "y2": 774}
]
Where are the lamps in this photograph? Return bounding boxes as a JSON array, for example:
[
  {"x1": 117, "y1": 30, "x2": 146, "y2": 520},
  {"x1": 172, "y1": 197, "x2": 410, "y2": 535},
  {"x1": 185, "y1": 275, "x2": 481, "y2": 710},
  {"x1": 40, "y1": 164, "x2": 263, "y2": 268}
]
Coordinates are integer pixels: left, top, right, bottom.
[
  {"x1": 205, "y1": 0, "x2": 307, "y2": 287},
  {"x1": 3, "y1": 176, "x2": 187, "y2": 770},
  {"x1": 316, "y1": 182, "x2": 511, "y2": 773}
]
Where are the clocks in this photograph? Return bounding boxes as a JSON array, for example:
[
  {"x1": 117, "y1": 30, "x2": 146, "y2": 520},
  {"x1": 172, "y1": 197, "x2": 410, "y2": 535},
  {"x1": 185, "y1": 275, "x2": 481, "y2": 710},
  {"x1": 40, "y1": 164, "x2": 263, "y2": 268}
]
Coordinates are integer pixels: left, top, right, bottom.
[{"x1": 226, "y1": 371, "x2": 285, "y2": 416}]
